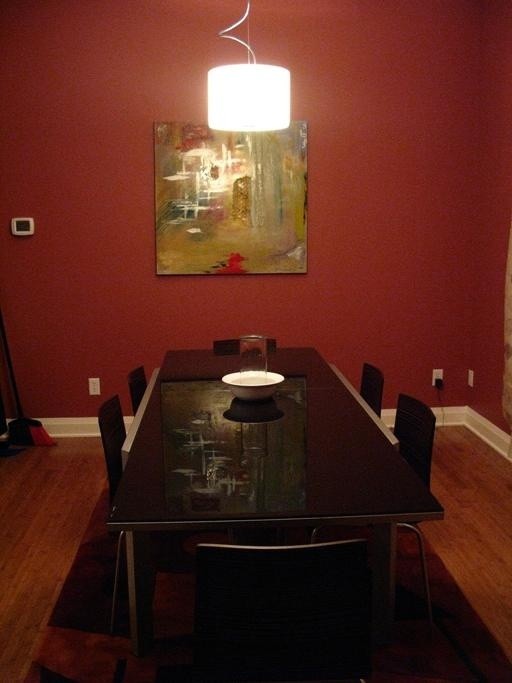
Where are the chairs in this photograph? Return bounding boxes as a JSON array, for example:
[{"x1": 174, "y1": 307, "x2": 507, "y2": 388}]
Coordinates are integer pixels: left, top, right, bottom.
[
  {"x1": 126, "y1": 362, "x2": 149, "y2": 422},
  {"x1": 212, "y1": 339, "x2": 277, "y2": 354},
  {"x1": 96, "y1": 392, "x2": 127, "y2": 646},
  {"x1": 391, "y1": 391, "x2": 437, "y2": 634},
  {"x1": 358, "y1": 361, "x2": 385, "y2": 419}
]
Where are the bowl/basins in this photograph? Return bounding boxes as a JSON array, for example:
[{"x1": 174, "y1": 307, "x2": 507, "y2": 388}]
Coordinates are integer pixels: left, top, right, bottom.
[{"x1": 221, "y1": 370, "x2": 285, "y2": 400}]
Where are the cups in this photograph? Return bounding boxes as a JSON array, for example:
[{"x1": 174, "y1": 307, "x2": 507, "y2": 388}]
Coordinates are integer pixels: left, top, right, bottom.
[{"x1": 240, "y1": 335, "x2": 267, "y2": 384}]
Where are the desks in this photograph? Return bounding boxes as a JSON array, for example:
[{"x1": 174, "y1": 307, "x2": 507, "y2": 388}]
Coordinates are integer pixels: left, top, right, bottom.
[{"x1": 96, "y1": 339, "x2": 447, "y2": 681}]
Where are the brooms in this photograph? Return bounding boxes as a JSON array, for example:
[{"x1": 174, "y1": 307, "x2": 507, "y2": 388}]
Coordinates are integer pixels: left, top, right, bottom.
[{"x1": 0, "y1": 313, "x2": 58, "y2": 448}]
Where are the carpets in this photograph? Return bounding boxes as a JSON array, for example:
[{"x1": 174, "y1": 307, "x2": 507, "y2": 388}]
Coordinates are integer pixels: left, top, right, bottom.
[{"x1": 20, "y1": 475, "x2": 512, "y2": 682}]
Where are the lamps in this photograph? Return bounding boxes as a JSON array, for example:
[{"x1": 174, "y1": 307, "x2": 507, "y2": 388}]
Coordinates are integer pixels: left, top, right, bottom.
[{"x1": 205, "y1": 0, "x2": 291, "y2": 133}]
